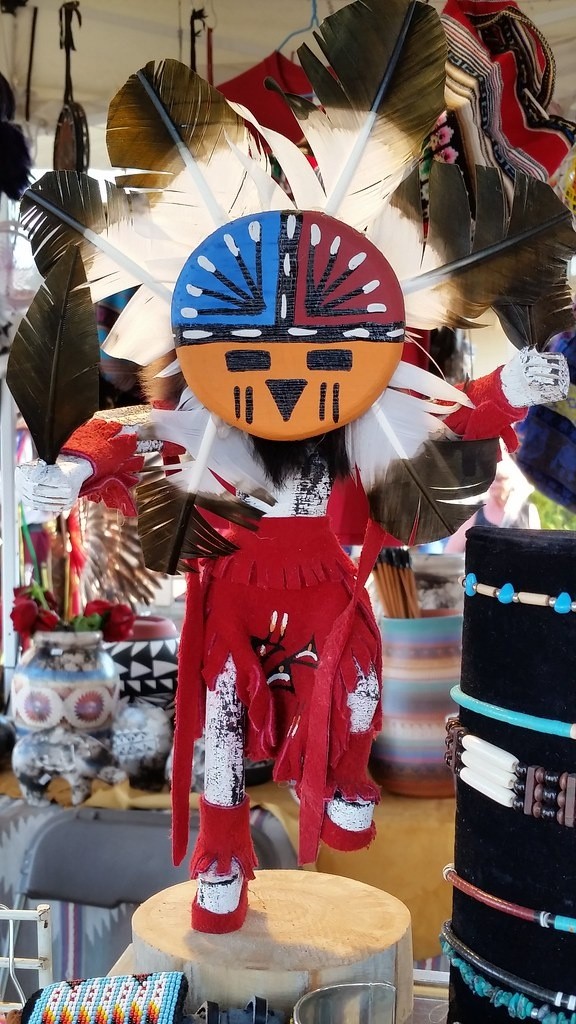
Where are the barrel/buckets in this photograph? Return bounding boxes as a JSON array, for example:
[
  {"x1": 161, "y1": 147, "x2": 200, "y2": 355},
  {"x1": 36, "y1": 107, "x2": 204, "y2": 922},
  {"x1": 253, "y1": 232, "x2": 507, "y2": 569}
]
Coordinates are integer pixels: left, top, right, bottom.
[{"x1": 375, "y1": 609, "x2": 463, "y2": 795}]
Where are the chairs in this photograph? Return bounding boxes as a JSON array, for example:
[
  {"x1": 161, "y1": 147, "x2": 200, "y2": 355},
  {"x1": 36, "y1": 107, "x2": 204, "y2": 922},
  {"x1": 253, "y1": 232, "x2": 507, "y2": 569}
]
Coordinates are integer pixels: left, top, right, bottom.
[{"x1": 1, "y1": 807, "x2": 297, "y2": 1001}]
